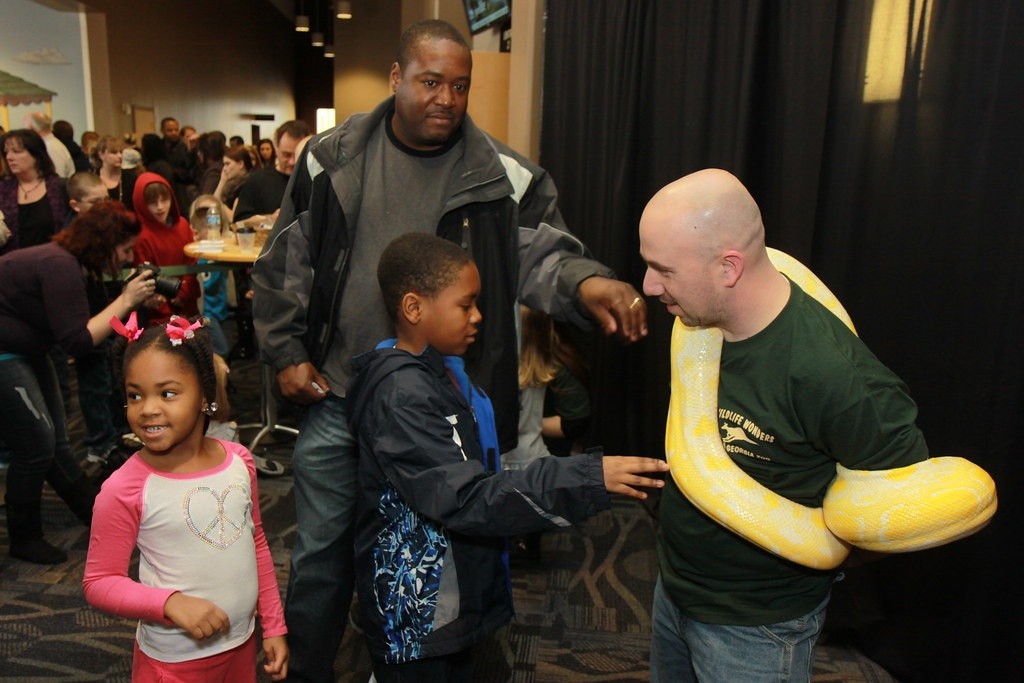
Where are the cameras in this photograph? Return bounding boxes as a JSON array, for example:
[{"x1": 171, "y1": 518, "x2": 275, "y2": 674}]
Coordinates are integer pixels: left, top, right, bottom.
[{"x1": 135, "y1": 261, "x2": 182, "y2": 299}]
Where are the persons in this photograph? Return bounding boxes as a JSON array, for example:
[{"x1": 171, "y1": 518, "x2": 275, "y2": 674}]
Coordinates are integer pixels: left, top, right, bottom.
[
  {"x1": 638, "y1": 167, "x2": 927, "y2": 683},
  {"x1": 82, "y1": 312, "x2": 291, "y2": 683},
  {"x1": 0, "y1": 112, "x2": 313, "y2": 561},
  {"x1": 497, "y1": 305, "x2": 591, "y2": 567},
  {"x1": 253, "y1": 17, "x2": 647, "y2": 683},
  {"x1": 346, "y1": 232, "x2": 669, "y2": 683}
]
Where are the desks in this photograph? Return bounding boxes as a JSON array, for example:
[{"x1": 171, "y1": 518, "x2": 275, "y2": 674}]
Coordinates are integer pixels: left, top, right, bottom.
[{"x1": 183, "y1": 234, "x2": 300, "y2": 454}]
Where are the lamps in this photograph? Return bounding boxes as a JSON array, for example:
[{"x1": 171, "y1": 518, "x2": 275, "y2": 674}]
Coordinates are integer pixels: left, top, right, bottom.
[
  {"x1": 295, "y1": 0, "x2": 310, "y2": 32},
  {"x1": 324, "y1": 0, "x2": 336, "y2": 58},
  {"x1": 336, "y1": 0, "x2": 352, "y2": 19},
  {"x1": 312, "y1": 0, "x2": 324, "y2": 47}
]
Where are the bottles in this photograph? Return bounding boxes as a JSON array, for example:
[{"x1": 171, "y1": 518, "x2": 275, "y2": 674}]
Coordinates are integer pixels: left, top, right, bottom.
[{"x1": 206, "y1": 203, "x2": 221, "y2": 242}]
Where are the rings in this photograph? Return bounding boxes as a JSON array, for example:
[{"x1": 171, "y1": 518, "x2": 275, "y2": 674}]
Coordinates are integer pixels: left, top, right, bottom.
[{"x1": 630, "y1": 297, "x2": 643, "y2": 309}]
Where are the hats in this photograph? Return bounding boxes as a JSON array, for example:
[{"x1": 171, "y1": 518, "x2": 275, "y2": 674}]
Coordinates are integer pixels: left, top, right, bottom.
[{"x1": 120, "y1": 147, "x2": 141, "y2": 169}]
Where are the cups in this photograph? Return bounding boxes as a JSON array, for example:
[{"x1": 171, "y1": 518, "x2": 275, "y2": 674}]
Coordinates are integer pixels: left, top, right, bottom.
[{"x1": 236, "y1": 227, "x2": 257, "y2": 254}]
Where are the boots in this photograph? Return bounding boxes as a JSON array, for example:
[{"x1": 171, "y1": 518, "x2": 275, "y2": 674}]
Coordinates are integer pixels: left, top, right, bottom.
[
  {"x1": 4, "y1": 499, "x2": 68, "y2": 564},
  {"x1": 61, "y1": 473, "x2": 100, "y2": 527}
]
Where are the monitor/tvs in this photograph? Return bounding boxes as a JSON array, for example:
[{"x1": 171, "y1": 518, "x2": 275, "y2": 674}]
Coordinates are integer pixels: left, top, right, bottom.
[{"x1": 462, "y1": 0, "x2": 511, "y2": 36}]
